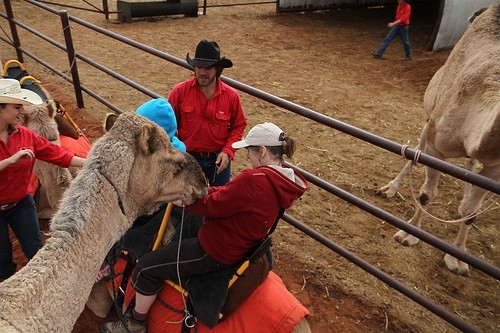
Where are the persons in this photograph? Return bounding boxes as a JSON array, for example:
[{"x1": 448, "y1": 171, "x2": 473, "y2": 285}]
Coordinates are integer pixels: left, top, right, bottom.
[
  {"x1": 100, "y1": 121, "x2": 310, "y2": 333},
  {"x1": 370, "y1": 1, "x2": 413, "y2": 61},
  {"x1": 168, "y1": 38, "x2": 249, "y2": 188},
  {"x1": 123, "y1": 97, "x2": 189, "y2": 156},
  {"x1": 0, "y1": 79, "x2": 87, "y2": 283}
]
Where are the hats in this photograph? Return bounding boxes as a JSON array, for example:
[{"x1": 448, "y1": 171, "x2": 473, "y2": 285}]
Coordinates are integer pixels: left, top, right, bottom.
[
  {"x1": 186, "y1": 39, "x2": 233, "y2": 68},
  {"x1": 0, "y1": 79, "x2": 43, "y2": 104},
  {"x1": 232, "y1": 122, "x2": 286, "y2": 148}
]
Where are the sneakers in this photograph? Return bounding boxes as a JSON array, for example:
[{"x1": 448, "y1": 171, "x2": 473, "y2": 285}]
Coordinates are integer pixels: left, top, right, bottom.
[
  {"x1": 401, "y1": 56, "x2": 411, "y2": 59},
  {"x1": 371, "y1": 52, "x2": 381, "y2": 58},
  {"x1": 101, "y1": 311, "x2": 147, "y2": 333}
]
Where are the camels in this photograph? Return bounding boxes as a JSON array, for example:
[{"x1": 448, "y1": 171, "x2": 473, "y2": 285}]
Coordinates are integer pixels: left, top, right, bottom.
[
  {"x1": 0, "y1": 101, "x2": 312, "y2": 332},
  {"x1": 376, "y1": 0, "x2": 500, "y2": 277}
]
